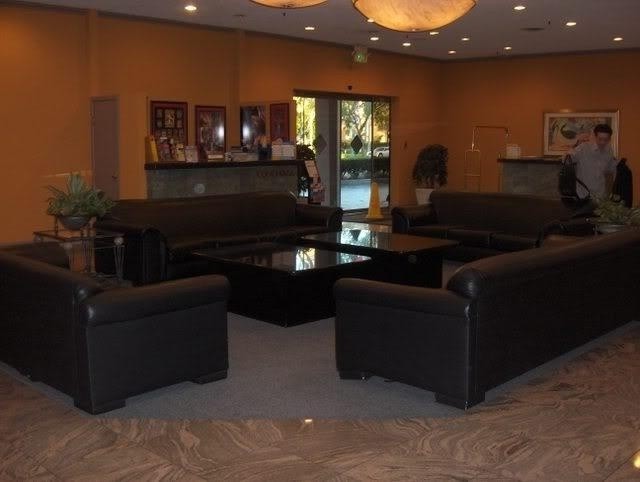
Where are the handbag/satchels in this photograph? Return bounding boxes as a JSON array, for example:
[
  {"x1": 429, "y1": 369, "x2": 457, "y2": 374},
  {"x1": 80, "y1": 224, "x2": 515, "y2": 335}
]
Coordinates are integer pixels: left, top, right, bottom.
[{"x1": 559, "y1": 155, "x2": 576, "y2": 196}]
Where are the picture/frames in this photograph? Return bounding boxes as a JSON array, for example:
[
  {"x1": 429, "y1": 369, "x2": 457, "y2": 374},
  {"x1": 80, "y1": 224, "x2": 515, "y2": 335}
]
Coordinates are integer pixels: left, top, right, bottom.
[
  {"x1": 270, "y1": 103, "x2": 290, "y2": 142},
  {"x1": 195, "y1": 105, "x2": 226, "y2": 156},
  {"x1": 240, "y1": 105, "x2": 266, "y2": 149},
  {"x1": 150, "y1": 100, "x2": 188, "y2": 147},
  {"x1": 542, "y1": 109, "x2": 619, "y2": 162}
]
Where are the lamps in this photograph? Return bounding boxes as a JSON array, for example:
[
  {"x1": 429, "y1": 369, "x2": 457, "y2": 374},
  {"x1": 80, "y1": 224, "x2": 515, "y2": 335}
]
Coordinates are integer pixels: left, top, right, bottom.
[
  {"x1": 352, "y1": 0, "x2": 476, "y2": 33},
  {"x1": 252, "y1": 0, "x2": 327, "y2": 9}
]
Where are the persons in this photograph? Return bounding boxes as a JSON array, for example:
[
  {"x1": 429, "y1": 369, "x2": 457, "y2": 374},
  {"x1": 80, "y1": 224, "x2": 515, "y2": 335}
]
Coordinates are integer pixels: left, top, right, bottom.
[
  {"x1": 559, "y1": 124, "x2": 616, "y2": 201},
  {"x1": 250, "y1": 118, "x2": 269, "y2": 153}
]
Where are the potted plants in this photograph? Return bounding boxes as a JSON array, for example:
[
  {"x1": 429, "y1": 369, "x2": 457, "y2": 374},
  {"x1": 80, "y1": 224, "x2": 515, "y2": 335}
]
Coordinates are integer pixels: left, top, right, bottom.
[
  {"x1": 413, "y1": 143, "x2": 450, "y2": 207},
  {"x1": 44, "y1": 171, "x2": 121, "y2": 231},
  {"x1": 588, "y1": 192, "x2": 639, "y2": 235},
  {"x1": 297, "y1": 143, "x2": 315, "y2": 206}
]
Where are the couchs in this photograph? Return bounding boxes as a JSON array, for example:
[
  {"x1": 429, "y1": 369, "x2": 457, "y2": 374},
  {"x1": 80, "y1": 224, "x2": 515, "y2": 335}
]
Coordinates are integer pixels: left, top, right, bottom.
[
  {"x1": 391, "y1": 191, "x2": 577, "y2": 262},
  {"x1": 1, "y1": 241, "x2": 232, "y2": 416},
  {"x1": 92, "y1": 190, "x2": 343, "y2": 286},
  {"x1": 333, "y1": 226, "x2": 639, "y2": 411}
]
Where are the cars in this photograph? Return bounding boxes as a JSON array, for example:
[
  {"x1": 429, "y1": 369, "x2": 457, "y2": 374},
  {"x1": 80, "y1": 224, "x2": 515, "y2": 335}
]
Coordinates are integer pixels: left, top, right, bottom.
[{"x1": 367, "y1": 146, "x2": 389, "y2": 158}]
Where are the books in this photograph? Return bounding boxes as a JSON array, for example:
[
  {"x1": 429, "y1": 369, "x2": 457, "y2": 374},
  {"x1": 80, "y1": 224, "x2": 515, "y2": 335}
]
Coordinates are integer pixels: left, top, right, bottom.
[{"x1": 144, "y1": 135, "x2": 199, "y2": 164}]
